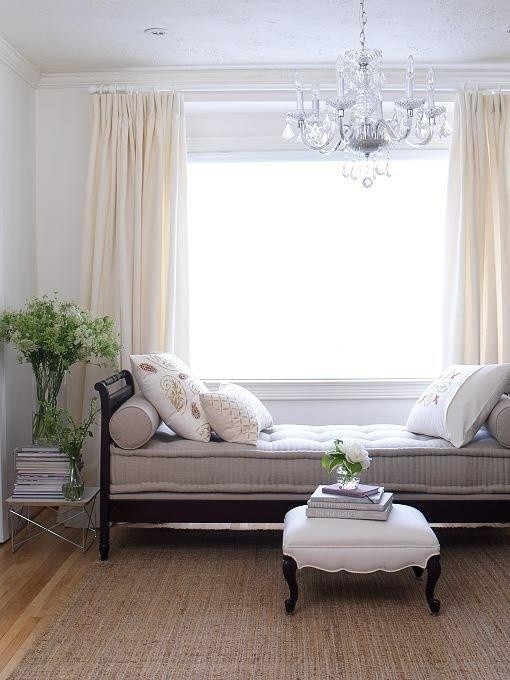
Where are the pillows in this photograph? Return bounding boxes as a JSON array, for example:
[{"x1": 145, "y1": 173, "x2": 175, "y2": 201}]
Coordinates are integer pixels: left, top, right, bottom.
[
  {"x1": 200, "y1": 381, "x2": 274, "y2": 445},
  {"x1": 131, "y1": 353, "x2": 211, "y2": 443},
  {"x1": 109, "y1": 391, "x2": 162, "y2": 449},
  {"x1": 484, "y1": 394, "x2": 510, "y2": 447},
  {"x1": 405, "y1": 364, "x2": 510, "y2": 449}
]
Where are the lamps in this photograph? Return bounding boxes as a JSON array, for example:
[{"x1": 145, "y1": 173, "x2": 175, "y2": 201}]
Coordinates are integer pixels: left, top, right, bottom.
[{"x1": 284, "y1": 0, "x2": 452, "y2": 188}]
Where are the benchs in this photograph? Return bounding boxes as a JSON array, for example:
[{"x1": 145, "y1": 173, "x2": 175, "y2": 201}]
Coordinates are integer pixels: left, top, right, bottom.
[{"x1": 93, "y1": 370, "x2": 510, "y2": 561}]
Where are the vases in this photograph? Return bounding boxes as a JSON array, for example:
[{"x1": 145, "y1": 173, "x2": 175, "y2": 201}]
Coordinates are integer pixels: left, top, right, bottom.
[
  {"x1": 337, "y1": 467, "x2": 360, "y2": 489},
  {"x1": 30, "y1": 368, "x2": 66, "y2": 447}
]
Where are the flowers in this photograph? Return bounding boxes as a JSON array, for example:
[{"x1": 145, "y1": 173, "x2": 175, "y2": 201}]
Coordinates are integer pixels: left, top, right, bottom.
[
  {"x1": 323, "y1": 437, "x2": 373, "y2": 485},
  {"x1": 0, "y1": 291, "x2": 122, "y2": 438}
]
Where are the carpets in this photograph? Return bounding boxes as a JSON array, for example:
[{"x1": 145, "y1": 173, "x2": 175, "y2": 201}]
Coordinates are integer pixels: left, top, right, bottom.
[{"x1": 8, "y1": 526, "x2": 510, "y2": 680}]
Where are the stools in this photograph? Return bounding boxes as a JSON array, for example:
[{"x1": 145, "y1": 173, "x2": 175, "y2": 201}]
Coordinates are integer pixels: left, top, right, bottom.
[{"x1": 282, "y1": 505, "x2": 442, "y2": 615}]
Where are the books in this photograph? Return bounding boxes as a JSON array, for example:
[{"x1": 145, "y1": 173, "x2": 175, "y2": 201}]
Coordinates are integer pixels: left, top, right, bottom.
[
  {"x1": 306, "y1": 505, "x2": 394, "y2": 521},
  {"x1": 312, "y1": 484, "x2": 385, "y2": 504},
  {"x1": 321, "y1": 483, "x2": 381, "y2": 499},
  {"x1": 12, "y1": 445, "x2": 85, "y2": 499},
  {"x1": 306, "y1": 492, "x2": 394, "y2": 512}
]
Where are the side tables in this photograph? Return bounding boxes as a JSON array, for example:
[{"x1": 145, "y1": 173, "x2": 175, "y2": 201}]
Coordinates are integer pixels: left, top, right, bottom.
[{"x1": 5, "y1": 487, "x2": 100, "y2": 553}]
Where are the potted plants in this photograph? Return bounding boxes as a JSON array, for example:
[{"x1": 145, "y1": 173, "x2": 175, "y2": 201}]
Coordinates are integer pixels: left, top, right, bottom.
[{"x1": 59, "y1": 397, "x2": 103, "y2": 502}]
